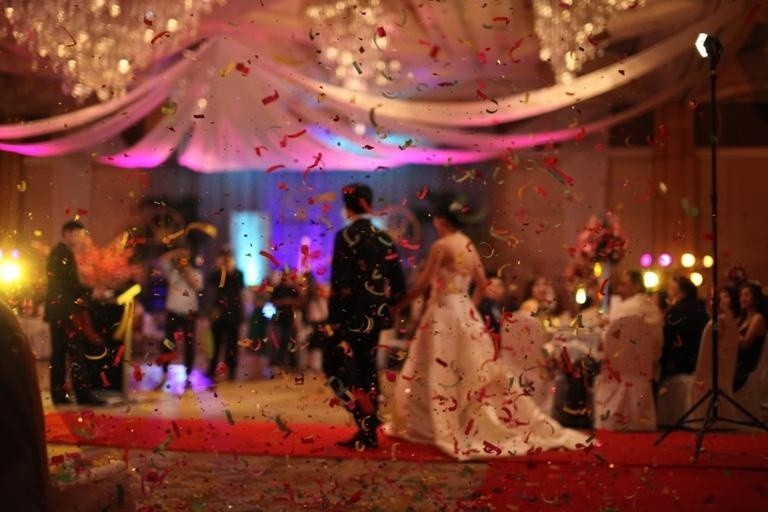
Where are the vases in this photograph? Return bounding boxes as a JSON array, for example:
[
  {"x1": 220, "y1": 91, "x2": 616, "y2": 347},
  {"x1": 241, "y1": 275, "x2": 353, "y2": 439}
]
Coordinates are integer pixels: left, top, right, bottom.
[{"x1": 599, "y1": 262, "x2": 609, "y2": 319}]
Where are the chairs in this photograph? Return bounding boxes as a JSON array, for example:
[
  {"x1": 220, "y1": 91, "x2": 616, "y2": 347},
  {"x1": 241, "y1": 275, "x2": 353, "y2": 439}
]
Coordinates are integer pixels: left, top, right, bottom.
[
  {"x1": 499, "y1": 310, "x2": 568, "y2": 418},
  {"x1": 661, "y1": 315, "x2": 739, "y2": 431},
  {"x1": 592, "y1": 312, "x2": 657, "y2": 432},
  {"x1": 1, "y1": 302, "x2": 134, "y2": 512}
]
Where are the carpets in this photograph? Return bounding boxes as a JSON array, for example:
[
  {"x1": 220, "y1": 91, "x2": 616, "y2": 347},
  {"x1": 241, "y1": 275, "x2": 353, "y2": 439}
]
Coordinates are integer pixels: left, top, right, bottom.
[{"x1": 41, "y1": 409, "x2": 767, "y2": 470}]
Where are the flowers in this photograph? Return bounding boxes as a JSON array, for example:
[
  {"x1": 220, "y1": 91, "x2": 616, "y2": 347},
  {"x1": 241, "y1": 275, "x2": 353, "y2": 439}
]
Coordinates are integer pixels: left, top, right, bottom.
[{"x1": 576, "y1": 211, "x2": 626, "y2": 261}]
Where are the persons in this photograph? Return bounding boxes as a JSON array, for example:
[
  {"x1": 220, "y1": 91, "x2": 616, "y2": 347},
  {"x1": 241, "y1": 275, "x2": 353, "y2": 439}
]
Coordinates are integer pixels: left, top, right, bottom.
[
  {"x1": 477, "y1": 272, "x2": 505, "y2": 333},
  {"x1": 719, "y1": 287, "x2": 742, "y2": 326},
  {"x1": 608, "y1": 269, "x2": 664, "y2": 366},
  {"x1": 206, "y1": 248, "x2": 244, "y2": 380},
  {"x1": 158, "y1": 245, "x2": 204, "y2": 375},
  {"x1": 42, "y1": 220, "x2": 109, "y2": 407},
  {"x1": 382, "y1": 201, "x2": 602, "y2": 461},
  {"x1": 322, "y1": 183, "x2": 406, "y2": 450},
  {"x1": 654, "y1": 276, "x2": 707, "y2": 397},
  {"x1": 519, "y1": 275, "x2": 548, "y2": 320},
  {"x1": 732, "y1": 284, "x2": 764, "y2": 394}
]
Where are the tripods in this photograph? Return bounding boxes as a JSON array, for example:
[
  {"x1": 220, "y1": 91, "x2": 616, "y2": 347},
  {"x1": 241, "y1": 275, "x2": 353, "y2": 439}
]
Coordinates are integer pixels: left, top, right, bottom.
[{"x1": 653, "y1": 51, "x2": 767, "y2": 464}]
